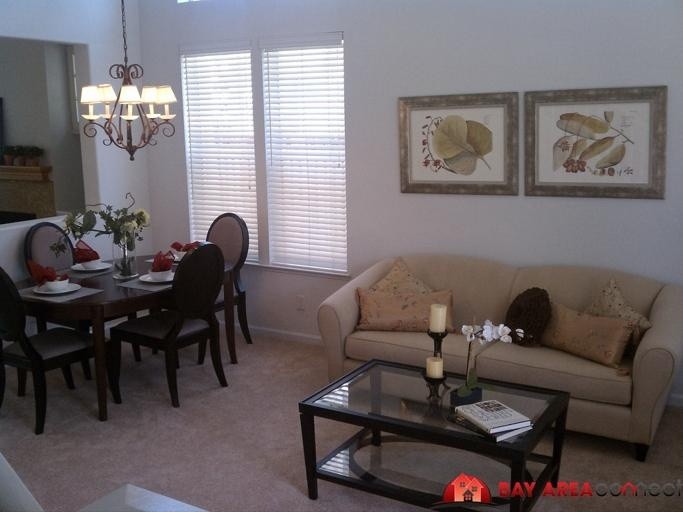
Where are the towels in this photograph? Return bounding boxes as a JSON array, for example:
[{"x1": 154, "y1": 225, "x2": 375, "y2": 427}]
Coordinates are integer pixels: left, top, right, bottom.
[
  {"x1": 170, "y1": 240, "x2": 202, "y2": 251},
  {"x1": 71, "y1": 240, "x2": 100, "y2": 264},
  {"x1": 151, "y1": 250, "x2": 176, "y2": 272},
  {"x1": 27, "y1": 258, "x2": 68, "y2": 288}
]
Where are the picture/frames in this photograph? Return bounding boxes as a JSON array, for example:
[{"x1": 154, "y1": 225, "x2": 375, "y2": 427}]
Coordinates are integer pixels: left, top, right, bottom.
[
  {"x1": 524, "y1": 83, "x2": 669, "y2": 199},
  {"x1": 397, "y1": 89, "x2": 520, "y2": 194}
]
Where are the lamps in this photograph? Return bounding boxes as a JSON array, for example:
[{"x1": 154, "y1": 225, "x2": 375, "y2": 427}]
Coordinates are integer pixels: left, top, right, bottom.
[{"x1": 78, "y1": 0, "x2": 178, "y2": 160}]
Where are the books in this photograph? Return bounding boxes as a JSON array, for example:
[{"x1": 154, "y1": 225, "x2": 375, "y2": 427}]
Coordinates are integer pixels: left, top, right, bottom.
[{"x1": 447, "y1": 399, "x2": 533, "y2": 443}]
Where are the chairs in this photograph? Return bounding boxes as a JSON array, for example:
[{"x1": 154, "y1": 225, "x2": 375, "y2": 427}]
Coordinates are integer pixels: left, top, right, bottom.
[
  {"x1": 110, "y1": 242, "x2": 228, "y2": 408},
  {"x1": 170, "y1": 213, "x2": 253, "y2": 365},
  {"x1": 23, "y1": 222, "x2": 142, "y2": 381},
  {"x1": 0, "y1": 265, "x2": 123, "y2": 435}
]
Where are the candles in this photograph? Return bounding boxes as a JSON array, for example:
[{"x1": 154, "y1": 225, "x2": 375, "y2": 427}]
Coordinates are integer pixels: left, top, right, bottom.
[
  {"x1": 425, "y1": 356, "x2": 445, "y2": 379},
  {"x1": 429, "y1": 302, "x2": 449, "y2": 332}
]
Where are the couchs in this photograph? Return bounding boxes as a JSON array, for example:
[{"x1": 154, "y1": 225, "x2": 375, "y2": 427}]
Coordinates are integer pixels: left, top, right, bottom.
[{"x1": 314, "y1": 252, "x2": 683, "y2": 462}]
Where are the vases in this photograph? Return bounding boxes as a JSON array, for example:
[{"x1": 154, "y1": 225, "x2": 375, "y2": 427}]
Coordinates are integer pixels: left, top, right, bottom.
[
  {"x1": 449, "y1": 386, "x2": 484, "y2": 407},
  {"x1": 111, "y1": 232, "x2": 140, "y2": 281}
]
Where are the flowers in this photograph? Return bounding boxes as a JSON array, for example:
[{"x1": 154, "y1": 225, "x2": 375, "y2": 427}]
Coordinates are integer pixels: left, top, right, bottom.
[
  {"x1": 453, "y1": 316, "x2": 535, "y2": 395},
  {"x1": 49, "y1": 192, "x2": 151, "y2": 275}
]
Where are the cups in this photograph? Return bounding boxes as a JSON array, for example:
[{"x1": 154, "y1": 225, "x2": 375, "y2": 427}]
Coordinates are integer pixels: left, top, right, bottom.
[
  {"x1": 148, "y1": 268, "x2": 171, "y2": 280},
  {"x1": 81, "y1": 258, "x2": 101, "y2": 269},
  {"x1": 174, "y1": 250, "x2": 187, "y2": 260},
  {"x1": 44, "y1": 276, "x2": 69, "y2": 292}
]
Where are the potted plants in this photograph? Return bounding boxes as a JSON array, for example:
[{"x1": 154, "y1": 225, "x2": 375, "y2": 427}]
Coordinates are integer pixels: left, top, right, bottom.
[{"x1": 3, "y1": 144, "x2": 45, "y2": 168}]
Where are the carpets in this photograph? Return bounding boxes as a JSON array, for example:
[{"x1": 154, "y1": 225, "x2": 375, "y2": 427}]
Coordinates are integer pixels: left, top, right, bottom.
[{"x1": 0, "y1": 321, "x2": 683, "y2": 512}]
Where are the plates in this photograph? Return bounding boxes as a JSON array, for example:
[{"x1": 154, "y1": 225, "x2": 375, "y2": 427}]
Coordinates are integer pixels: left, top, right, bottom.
[
  {"x1": 32, "y1": 283, "x2": 81, "y2": 295},
  {"x1": 139, "y1": 272, "x2": 174, "y2": 283},
  {"x1": 173, "y1": 255, "x2": 181, "y2": 262},
  {"x1": 70, "y1": 263, "x2": 112, "y2": 271}
]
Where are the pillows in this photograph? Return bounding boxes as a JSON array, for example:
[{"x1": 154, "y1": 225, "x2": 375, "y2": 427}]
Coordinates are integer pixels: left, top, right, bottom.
[
  {"x1": 583, "y1": 277, "x2": 652, "y2": 349},
  {"x1": 370, "y1": 258, "x2": 432, "y2": 295},
  {"x1": 540, "y1": 298, "x2": 637, "y2": 368},
  {"x1": 501, "y1": 286, "x2": 552, "y2": 348},
  {"x1": 356, "y1": 287, "x2": 456, "y2": 338}
]
